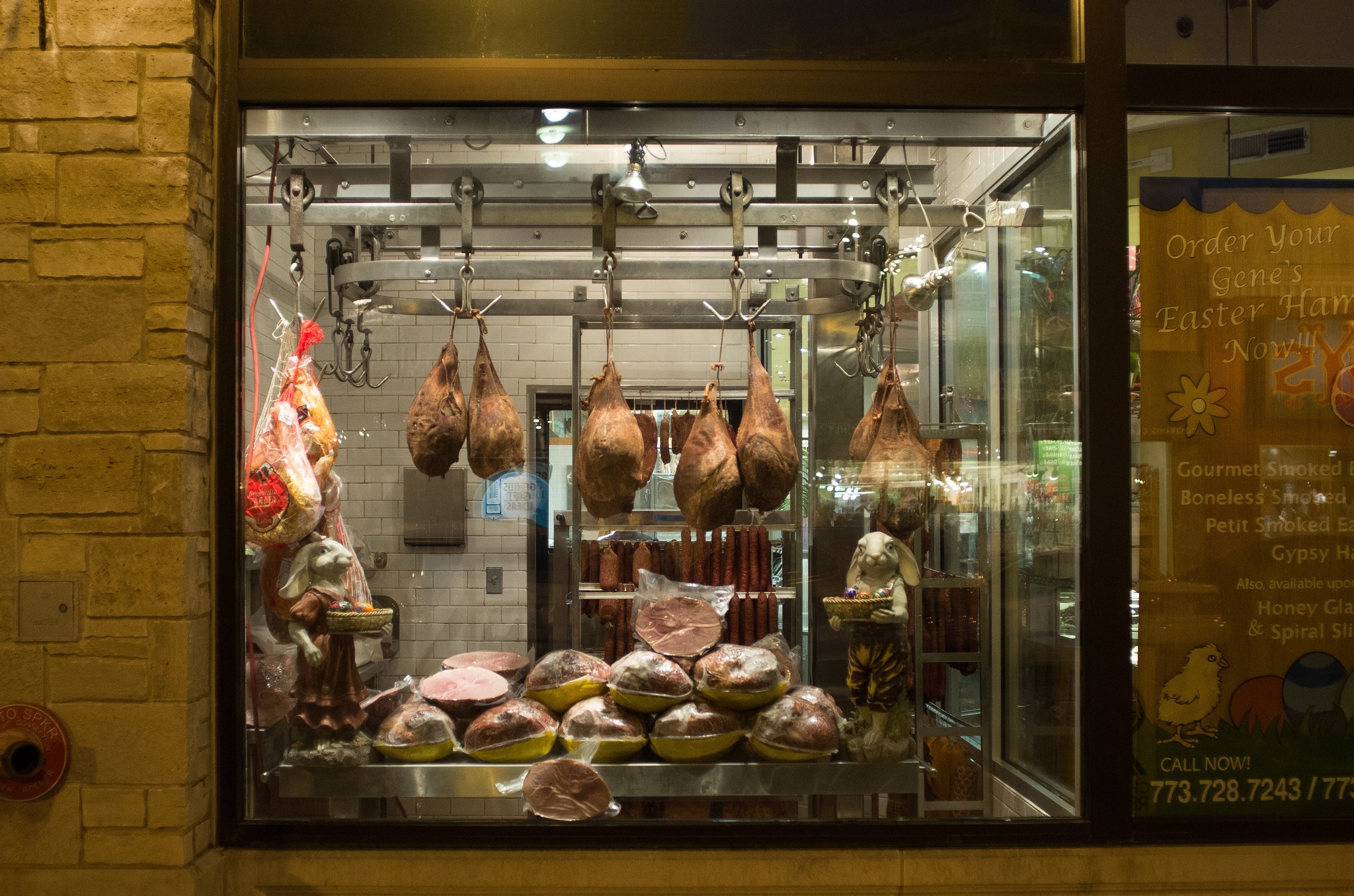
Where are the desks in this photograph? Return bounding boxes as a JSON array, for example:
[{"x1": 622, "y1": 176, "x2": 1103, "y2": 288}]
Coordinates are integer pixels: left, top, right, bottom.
[{"x1": 276, "y1": 754, "x2": 919, "y2": 821}]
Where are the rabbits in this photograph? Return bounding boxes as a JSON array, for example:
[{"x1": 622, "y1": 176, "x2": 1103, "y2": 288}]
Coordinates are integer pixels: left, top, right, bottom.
[{"x1": 829, "y1": 532, "x2": 922, "y2": 746}]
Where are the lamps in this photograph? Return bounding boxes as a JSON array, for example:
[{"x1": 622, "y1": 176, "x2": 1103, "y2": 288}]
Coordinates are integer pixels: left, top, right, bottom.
[
  {"x1": 901, "y1": 198, "x2": 985, "y2": 311},
  {"x1": 610, "y1": 136, "x2": 666, "y2": 203}
]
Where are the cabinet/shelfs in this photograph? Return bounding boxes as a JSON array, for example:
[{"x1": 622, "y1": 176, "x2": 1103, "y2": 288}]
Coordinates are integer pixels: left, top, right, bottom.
[
  {"x1": 914, "y1": 421, "x2": 993, "y2": 821},
  {"x1": 554, "y1": 316, "x2": 802, "y2": 687}
]
